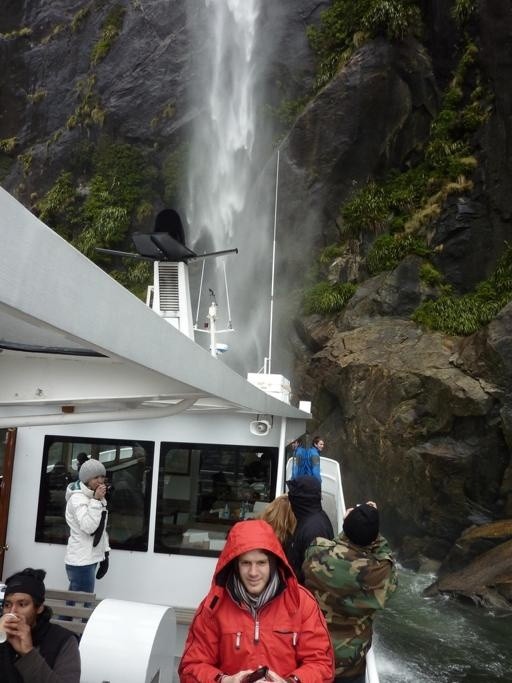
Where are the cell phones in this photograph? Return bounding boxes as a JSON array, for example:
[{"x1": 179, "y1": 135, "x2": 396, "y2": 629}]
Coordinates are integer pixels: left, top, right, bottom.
[{"x1": 242, "y1": 666, "x2": 269, "y2": 683}]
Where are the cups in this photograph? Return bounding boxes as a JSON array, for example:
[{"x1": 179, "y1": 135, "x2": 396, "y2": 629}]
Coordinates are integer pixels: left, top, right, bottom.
[{"x1": 0, "y1": 612, "x2": 18, "y2": 643}]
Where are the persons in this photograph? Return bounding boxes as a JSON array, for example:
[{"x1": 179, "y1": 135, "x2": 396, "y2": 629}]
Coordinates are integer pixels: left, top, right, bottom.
[
  {"x1": 57, "y1": 457, "x2": 112, "y2": 637},
  {"x1": 301, "y1": 435, "x2": 326, "y2": 482},
  {"x1": 77, "y1": 450, "x2": 89, "y2": 471},
  {"x1": 1, "y1": 567, "x2": 83, "y2": 682},
  {"x1": 252, "y1": 493, "x2": 296, "y2": 548},
  {"x1": 301, "y1": 500, "x2": 399, "y2": 682},
  {"x1": 288, "y1": 437, "x2": 303, "y2": 457},
  {"x1": 177, "y1": 519, "x2": 336, "y2": 682},
  {"x1": 45, "y1": 458, "x2": 74, "y2": 516},
  {"x1": 282, "y1": 474, "x2": 336, "y2": 584}
]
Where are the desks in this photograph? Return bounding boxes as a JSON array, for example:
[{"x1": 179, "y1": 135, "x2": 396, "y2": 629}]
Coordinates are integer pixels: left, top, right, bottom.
[{"x1": 159, "y1": 498, "x2": 193, "y2": 524}]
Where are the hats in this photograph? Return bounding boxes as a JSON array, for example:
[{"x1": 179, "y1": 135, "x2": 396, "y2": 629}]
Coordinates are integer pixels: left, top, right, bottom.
[
  {"x1": 4, "y1": 567, "x2": 45, "y2": 608},
  {"x1": 343, "y1": 504, "x2": 379, "y2": 546},
  {"x1": 77, "y1": 460, "x2": 106, "y2": 484}
]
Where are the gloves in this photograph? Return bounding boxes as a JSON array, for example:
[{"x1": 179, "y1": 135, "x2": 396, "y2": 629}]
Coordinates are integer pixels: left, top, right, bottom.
[
  {"x1": 89, "y1": 511, "x2": 107, "y2": 547},
  {"x1": 96, "y1": 552, "x2": 109, "y2": 579}
]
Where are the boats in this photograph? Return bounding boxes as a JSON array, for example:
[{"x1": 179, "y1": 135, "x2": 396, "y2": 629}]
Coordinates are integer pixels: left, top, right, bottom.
[{"x1": 0, "y1": 137, "x2": 390, "y2": 682}]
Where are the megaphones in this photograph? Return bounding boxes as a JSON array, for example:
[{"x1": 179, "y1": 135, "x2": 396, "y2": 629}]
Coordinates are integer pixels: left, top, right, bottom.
[{"x1": 249, "y1": 420, "x2": 272, "y2": 437}]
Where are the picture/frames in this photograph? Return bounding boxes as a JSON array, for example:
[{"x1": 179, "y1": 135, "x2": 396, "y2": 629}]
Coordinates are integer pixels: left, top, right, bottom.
[{"x1": 163, "y1": 448, "x2": 192, "y2": 476}]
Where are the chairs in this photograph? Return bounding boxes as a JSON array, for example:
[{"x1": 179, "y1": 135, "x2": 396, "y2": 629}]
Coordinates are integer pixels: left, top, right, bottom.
[{"x1": 42, "y1": 590, "x2": 96, "y2": 643}]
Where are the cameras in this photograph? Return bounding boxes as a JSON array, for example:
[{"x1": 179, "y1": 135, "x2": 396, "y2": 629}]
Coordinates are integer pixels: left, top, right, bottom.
[{"x1": 103, "y1": 482, "x2": 113, "y2": 492}]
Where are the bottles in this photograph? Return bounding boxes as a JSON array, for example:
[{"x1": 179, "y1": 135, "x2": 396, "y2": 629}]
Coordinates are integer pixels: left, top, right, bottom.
[{"x1": 224, "y1": 503, "x2": 230, "y2": 519}]
